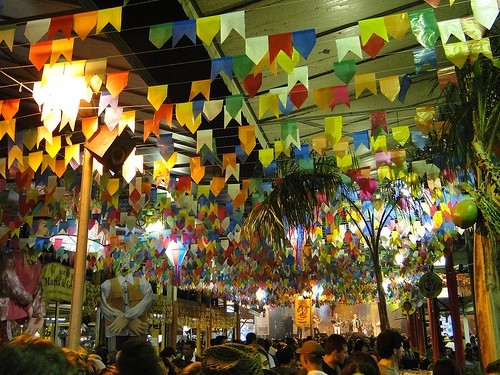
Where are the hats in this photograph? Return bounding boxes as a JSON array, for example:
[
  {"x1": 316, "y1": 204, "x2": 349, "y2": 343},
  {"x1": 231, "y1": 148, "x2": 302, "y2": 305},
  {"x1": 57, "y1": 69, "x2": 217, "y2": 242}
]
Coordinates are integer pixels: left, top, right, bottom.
[
  {"x1": 294, "y1": 340, "x2": 323, "y2": 357},
  {"x1": 200, "y1": 342, "x2": 263, "y2": 374}
]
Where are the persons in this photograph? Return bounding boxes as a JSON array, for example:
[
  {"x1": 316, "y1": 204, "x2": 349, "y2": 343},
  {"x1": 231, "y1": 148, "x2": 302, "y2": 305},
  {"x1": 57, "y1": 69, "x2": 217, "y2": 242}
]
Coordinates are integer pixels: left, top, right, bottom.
[
  {"x1": 0, "y1": 201, "x2": 54, "y2": 339},
  {"x1": 331, "y1": 313, "x2": 341, "y2": 335},
  {"x1": 0, "y1": 329, "x2": 500, "y2": 375},
  {"x1": 284, "y1": 316, "x2": 294, "y2": 337},
  {"x1": 98, "y1": 261, "x2": 154, "y2": 361},
  {"x1": 351, "y1": 314, "x2": 363, "y2": 332},
  {"x1": 367, "y1": 321, "x2": 374, "y2": 336},
  {"x1": 311, "y1": 315, "x2": 321, "y2": 335}
]
